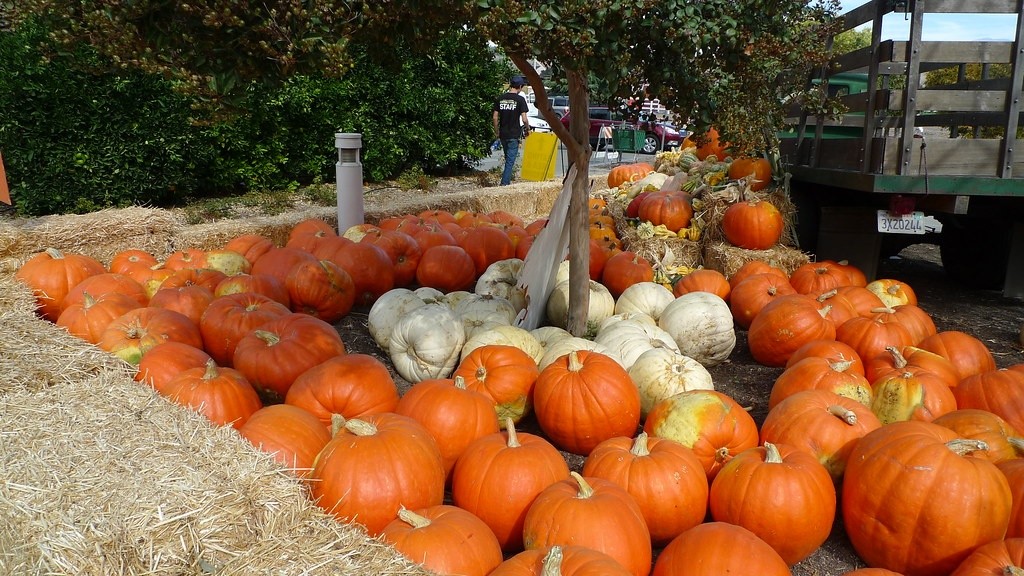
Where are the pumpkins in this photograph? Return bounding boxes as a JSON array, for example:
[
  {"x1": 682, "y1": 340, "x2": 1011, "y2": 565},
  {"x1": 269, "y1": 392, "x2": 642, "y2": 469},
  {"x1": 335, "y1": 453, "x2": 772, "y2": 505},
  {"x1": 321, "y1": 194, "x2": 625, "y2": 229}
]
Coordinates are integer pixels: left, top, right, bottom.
[{"x1": 20, "y1": 130, "x2": 1024, "y2": 576}]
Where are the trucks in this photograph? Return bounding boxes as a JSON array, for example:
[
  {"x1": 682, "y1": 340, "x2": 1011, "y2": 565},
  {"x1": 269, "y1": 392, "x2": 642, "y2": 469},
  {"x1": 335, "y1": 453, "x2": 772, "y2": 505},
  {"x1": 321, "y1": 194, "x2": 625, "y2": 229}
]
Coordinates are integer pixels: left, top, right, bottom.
[{"x1": 755, "y1": 0, "x2": 1024, "y2": 280}]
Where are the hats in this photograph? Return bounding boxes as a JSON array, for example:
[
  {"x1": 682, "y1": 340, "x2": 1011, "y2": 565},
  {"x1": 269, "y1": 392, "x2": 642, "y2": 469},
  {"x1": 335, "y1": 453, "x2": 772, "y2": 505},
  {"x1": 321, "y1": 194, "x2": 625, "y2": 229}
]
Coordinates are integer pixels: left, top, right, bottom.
[{"x1": 512, "y1": 76, "x2": 526, "y2": 86}]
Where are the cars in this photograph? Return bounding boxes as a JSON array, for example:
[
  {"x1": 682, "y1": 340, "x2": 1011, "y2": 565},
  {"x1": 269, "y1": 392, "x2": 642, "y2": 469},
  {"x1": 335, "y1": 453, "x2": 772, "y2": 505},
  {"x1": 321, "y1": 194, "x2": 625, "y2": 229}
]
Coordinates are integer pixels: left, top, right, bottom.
[
  {"x1": 560, "y1": 104, "x2": 688, "y2": 154},
  {"x1": 519, "y1": 103, "x2": 551, "y2": 137}
]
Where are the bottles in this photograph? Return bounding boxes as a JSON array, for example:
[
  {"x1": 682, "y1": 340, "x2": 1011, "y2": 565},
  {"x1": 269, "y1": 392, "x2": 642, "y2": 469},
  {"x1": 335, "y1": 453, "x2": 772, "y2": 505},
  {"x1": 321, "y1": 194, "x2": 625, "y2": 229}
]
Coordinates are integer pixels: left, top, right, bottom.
[{"x1": 490, "y1": 138, "x2": 502, "y2": 151}]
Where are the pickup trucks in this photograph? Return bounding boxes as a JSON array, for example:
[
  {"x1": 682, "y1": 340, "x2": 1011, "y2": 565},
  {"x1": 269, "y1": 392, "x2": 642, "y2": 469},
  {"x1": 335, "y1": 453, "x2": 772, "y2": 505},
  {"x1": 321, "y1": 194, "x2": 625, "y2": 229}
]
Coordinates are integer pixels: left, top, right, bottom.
[{"x1": 538, "y1": 96, "x2": 570, "y2": 119}]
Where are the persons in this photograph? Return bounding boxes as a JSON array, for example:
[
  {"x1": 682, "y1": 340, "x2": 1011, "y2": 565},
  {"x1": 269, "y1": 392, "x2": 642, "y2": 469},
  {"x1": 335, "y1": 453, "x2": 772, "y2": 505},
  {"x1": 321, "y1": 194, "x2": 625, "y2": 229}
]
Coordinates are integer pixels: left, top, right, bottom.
[{"x1": 493, "y1": 76, "x2": 529, "y2": 186}]
